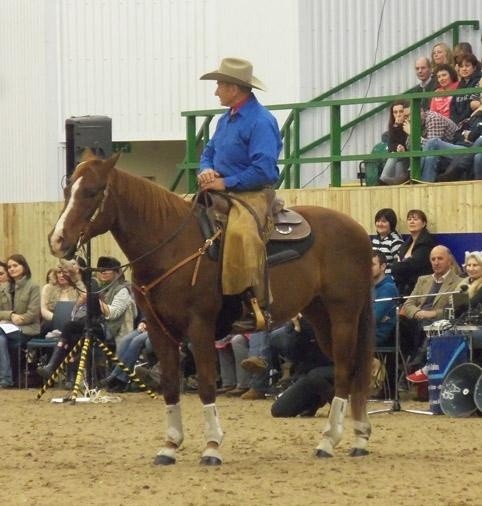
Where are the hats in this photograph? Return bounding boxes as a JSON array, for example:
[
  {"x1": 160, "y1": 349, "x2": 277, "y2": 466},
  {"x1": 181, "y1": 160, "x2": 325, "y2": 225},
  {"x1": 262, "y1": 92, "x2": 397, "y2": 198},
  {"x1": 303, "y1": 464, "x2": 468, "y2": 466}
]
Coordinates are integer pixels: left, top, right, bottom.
[{"x1": 199, "y1": 56, "x2": 267, "y2": 92}]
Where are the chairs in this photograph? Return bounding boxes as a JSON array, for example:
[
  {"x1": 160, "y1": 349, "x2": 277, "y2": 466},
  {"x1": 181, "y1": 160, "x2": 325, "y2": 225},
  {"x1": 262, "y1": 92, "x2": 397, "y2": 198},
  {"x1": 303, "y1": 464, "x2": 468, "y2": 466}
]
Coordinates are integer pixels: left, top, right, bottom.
[
  {"x1": 8, "y1": 301, "x2": 116, "y2": 388},
  {"x1": 372, "y1": 346, "x2": 410, "y2": 400}
]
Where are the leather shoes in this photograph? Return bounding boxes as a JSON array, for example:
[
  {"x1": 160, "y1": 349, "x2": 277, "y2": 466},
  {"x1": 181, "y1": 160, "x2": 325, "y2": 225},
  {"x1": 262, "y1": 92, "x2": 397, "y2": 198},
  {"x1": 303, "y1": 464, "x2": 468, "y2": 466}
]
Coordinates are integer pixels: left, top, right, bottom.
[
  {"x1": 231, "y1": 311, "x2": 271, "y2": 333},
  {"x1": 100, "y1": 375, "x2": 123, "y2": 393}
]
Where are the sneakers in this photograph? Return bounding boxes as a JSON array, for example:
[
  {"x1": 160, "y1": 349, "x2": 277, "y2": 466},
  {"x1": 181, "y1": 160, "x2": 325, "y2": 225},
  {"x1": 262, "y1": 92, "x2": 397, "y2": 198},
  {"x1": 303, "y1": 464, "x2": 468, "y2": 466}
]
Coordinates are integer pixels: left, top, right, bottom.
[
  {"x1": 215, "y1": 385, "x2": 236, "y2": 394},
  {"x1": 240, "y1": 356, "x2": 269, "y2": 377},
  {"x1": 226, "y1": 385, "x2": 249, "y2": 398},
  {"x1": 404, "y1": 367, "x2": 427, "y2": 384},
  {"x1": 135, "y1": 367, "x2": 163, "y2": 396},
  {"x1": 240, "y1": 388, "x2": 267, "y2": 401}
]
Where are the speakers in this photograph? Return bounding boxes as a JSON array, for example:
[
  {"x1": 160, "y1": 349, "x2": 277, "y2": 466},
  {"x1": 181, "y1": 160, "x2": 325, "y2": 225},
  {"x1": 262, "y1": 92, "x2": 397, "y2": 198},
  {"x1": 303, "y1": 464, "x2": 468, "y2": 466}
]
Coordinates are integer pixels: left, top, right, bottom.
[{"x1": 66, "y1": 115, "x2": 112, "y2": 182}]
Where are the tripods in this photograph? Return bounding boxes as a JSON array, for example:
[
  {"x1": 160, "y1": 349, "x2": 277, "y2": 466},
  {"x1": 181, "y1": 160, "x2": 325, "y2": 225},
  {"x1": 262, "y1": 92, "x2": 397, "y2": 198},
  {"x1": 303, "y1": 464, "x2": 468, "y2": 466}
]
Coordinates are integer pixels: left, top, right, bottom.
[
  {"x1": 34, "y1": 237, "x2": 159, "y2": 405},
  {"x1": 367, "y1": 288, "x2": 458, "y2": 416}
]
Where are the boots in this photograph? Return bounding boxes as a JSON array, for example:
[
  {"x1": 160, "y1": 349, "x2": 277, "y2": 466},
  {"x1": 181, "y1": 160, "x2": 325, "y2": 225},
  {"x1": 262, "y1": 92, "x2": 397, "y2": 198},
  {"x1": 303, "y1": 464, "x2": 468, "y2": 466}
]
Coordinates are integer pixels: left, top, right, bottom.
[
  {"x1": 36, "y1": 335, "x2": 75, "y2": 384},
  {"x1": 65, "y1": 360, "x2": 78, "y2": 389}
]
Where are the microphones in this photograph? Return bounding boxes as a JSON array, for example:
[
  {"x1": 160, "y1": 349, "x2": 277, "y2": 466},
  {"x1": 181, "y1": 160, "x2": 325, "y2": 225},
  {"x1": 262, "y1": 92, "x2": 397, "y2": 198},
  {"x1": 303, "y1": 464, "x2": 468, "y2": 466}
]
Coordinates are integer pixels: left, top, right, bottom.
[{"x1": 460, "y1": 284, "x2": 469, "y2": 293}]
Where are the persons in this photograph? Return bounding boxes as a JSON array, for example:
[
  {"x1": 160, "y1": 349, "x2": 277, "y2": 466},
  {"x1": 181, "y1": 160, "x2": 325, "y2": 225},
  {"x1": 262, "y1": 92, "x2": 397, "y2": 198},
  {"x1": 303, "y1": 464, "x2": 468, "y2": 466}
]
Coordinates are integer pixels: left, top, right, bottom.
[
  {"x1": 371, "y1": 208, "x2": 480, "y2": 399},
  {"x1": 380, "y1": 44, "x2": 482, "y2": 184},
  {"x1": 1, "y1": 255, "x2": 163, "y2": 395},
  {"x1": 178, "y1": 312, "x2": 336, "y2": 418},
  {"x1": 199, "y1": 59, "x2": 282, "y2": 334}
]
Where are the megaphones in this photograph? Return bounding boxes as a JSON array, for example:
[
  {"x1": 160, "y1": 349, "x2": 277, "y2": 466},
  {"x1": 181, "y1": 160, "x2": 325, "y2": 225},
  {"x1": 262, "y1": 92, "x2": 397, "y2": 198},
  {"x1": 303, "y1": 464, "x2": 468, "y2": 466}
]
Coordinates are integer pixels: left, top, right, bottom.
[{"x1": 440, "y1": 363, "x2": 482, "y2": 418}]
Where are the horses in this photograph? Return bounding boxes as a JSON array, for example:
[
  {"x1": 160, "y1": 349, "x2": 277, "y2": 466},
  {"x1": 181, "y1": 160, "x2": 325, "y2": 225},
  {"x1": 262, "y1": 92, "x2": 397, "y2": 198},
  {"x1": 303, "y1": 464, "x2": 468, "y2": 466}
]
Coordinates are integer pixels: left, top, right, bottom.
[{"x1": 48, "y1": 145, "x2": 376, "y2": 472}]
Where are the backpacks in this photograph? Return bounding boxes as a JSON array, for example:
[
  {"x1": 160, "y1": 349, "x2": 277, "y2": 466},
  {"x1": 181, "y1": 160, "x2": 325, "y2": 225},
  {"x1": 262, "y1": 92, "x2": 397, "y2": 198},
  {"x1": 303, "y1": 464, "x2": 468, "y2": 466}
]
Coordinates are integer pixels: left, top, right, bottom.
[{"x1": 74, "y1": 300, "x2": 106, "y2": 331}]
